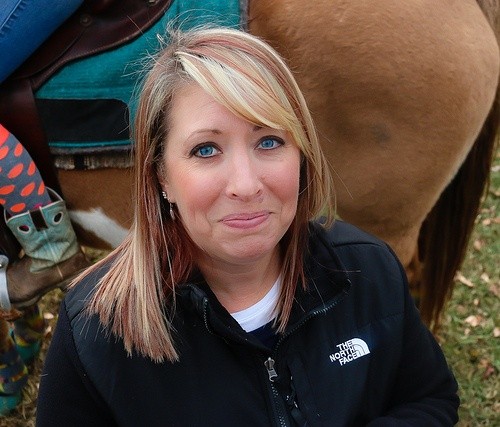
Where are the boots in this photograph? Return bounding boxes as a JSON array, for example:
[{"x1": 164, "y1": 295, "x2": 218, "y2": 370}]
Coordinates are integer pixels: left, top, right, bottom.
[{"x1": 4, "y1": 184, "x2": 85, "y2": 306}]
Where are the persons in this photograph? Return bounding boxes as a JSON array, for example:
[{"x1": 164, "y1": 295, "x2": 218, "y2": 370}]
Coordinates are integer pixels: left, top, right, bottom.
[
  {"x1": 0, "y1": 0, "x2": 92, "y2": 309},
  {"x1": 34, "y1": 22, "x2": 460, "y2": 427}
]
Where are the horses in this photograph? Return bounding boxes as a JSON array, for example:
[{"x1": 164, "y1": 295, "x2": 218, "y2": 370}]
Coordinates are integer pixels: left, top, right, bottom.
[{"x1": 1, "y1": 0, "x2": 500, "y2": 417}]
